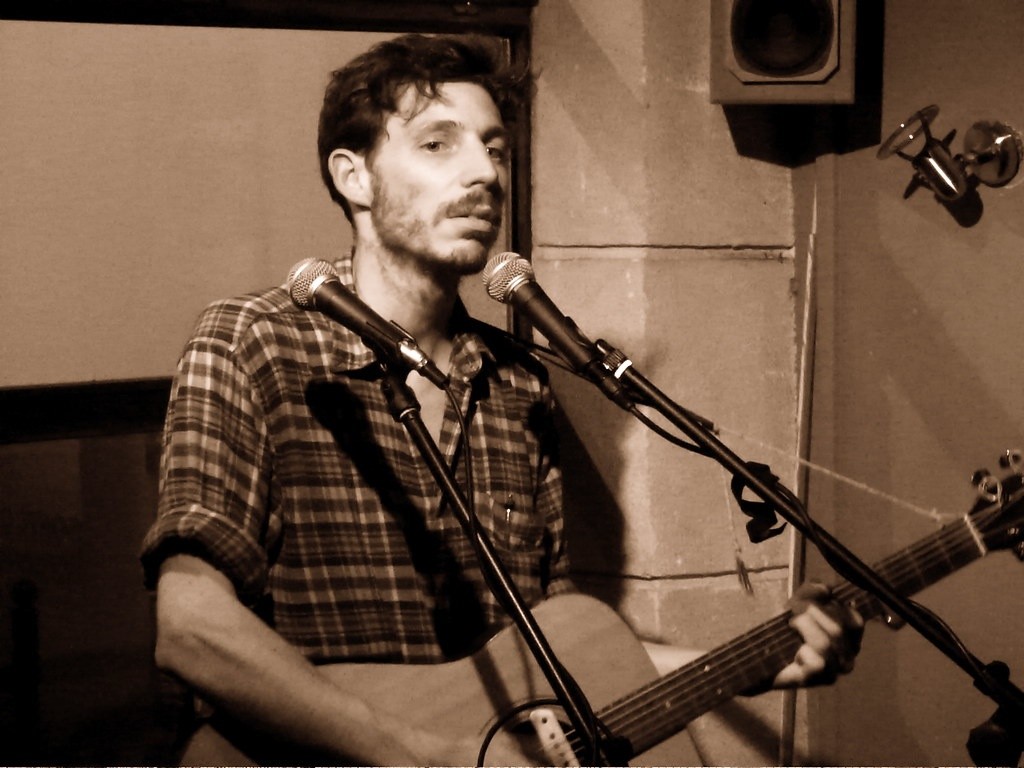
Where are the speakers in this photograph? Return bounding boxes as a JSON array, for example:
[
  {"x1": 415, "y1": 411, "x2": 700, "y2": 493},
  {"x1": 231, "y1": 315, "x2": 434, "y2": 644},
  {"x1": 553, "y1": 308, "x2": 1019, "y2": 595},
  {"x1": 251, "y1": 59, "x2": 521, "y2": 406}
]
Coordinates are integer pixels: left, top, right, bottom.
[{"x1": 707, "y1": 0, "x2": 859, "y2": 104}]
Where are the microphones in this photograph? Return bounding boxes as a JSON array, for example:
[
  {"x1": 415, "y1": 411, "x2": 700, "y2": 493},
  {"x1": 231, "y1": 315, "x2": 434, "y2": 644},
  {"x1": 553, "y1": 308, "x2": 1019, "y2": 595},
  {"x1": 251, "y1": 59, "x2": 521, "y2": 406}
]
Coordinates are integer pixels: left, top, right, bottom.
[
  {"x1": 287, "y1": 258, "x2": 450, "y2": 391},
  {"x1": 482, "y1": 251, "x2": 634, "y2": 411}
]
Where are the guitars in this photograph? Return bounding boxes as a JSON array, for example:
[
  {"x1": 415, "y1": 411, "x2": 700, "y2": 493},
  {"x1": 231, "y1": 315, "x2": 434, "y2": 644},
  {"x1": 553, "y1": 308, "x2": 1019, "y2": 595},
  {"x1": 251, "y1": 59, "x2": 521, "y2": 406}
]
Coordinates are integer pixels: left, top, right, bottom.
[{"x1": 179, "y1": 446, "x2": 1024, "y2": 766}]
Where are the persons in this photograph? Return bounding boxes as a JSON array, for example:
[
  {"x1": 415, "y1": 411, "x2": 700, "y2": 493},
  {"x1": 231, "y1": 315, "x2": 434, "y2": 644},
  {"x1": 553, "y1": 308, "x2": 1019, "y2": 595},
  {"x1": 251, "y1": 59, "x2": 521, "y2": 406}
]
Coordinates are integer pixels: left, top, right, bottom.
[{"x1": 139, "y1": 33, "x2": 866, "y2": 768}]
